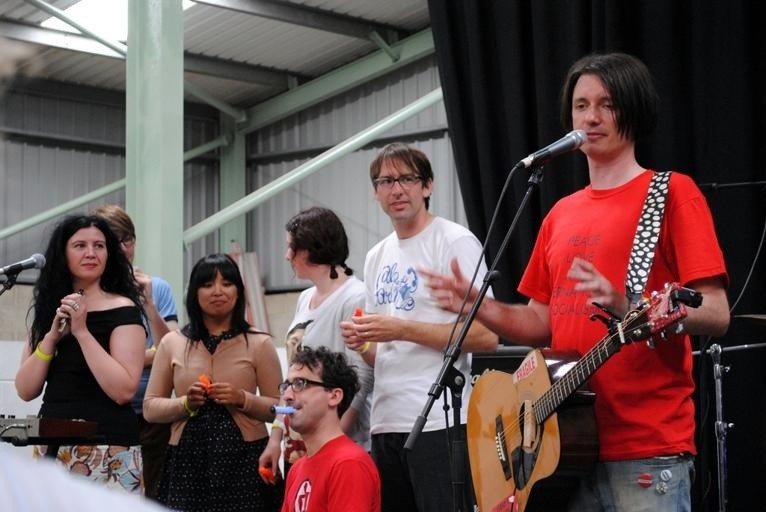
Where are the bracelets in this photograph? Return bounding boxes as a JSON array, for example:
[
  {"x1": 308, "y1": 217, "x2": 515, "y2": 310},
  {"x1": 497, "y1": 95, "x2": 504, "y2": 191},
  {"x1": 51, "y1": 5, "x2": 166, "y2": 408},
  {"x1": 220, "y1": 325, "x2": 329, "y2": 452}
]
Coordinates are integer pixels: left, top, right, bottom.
[
  {"x1": 270, "y1": 422, "x2": 283, "y2": 431},
  {"x1": 34, "y1": 343, "x2": 59, "y2": 360},
  {"x1": 356, "y1": 334, "x2": 369, "y2": 358},
  {"x1": 184, "y1": 398, "x2": 200, "y2": 418}
]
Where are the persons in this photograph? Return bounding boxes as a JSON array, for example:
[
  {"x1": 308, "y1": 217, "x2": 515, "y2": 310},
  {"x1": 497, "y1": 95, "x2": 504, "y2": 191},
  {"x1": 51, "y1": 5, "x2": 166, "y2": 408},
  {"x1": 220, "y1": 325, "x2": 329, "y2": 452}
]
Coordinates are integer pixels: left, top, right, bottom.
[
  {"x1": 256, "y1": 205, "x2": 374, "y2": 485},
  {"x1": 402, "y1": 48, "x2": 731, "y2": 512},
  {"x1": 272, "y1": 346, "x2": 384, "y2": 511},
  {"x1": 91, "y1": 204, "x2": 179, "y2": 418},
  {"x1": 139, "y1": 250, "x2": 283, "y2": 512},
  {"x1": 337, "y1": 140, "x2": 501, "y2": 512},
  {"x1": 14, "y1": 213, "x2": 146, "y2": 448}
]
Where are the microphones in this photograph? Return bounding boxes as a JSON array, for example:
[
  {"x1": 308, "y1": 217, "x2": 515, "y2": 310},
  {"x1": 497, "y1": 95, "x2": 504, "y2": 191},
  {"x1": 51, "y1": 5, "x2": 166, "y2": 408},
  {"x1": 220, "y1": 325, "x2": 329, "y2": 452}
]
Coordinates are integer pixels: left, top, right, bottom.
[
  {"x1": 516, "y1": 130, "x2": 587, "y2": 168},
  {"x1": 0, "y1": 253, "x2": 46, "y2": 274}
]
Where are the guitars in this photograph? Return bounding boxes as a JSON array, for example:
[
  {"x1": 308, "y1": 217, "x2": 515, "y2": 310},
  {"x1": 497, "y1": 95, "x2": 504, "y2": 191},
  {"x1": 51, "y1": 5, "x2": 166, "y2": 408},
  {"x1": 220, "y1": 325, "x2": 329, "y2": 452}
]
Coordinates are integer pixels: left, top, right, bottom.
[{"x1": 467, "y1": 282, "x2": 704, "y2": 512}]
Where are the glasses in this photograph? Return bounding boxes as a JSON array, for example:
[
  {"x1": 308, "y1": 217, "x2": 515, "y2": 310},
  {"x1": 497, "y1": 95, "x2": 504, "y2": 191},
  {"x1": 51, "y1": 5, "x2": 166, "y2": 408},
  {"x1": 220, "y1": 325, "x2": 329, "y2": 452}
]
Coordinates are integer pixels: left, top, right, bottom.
[
  {"x1": 119, "y1": 233, "x2": 136, "y2": 247},
  {"x1": 277, "y1": 378, "x2": 333, "y2": 396},
  {"x1": 372, "y1": 173, "x2": 424, "y2": 191}
]
